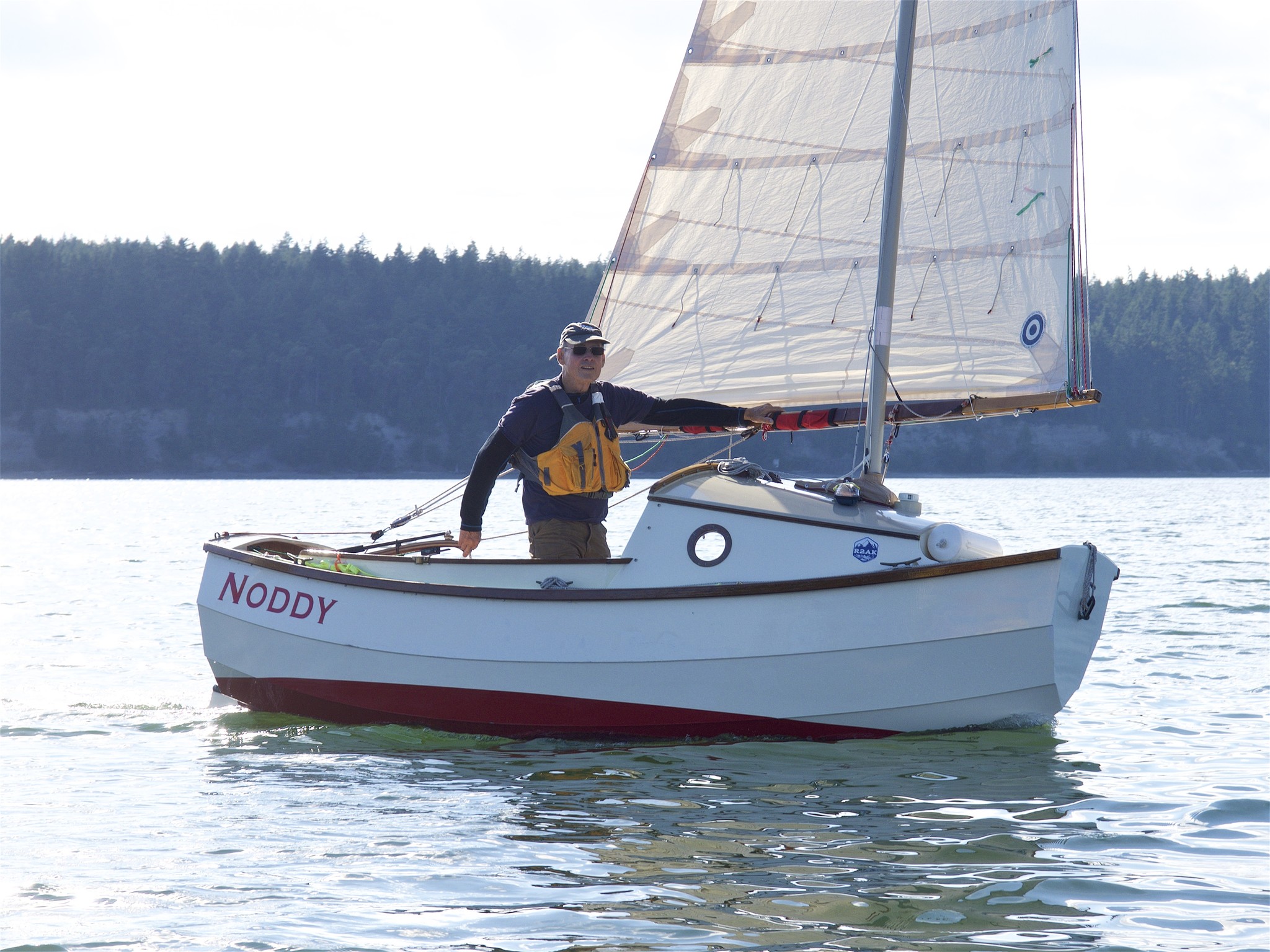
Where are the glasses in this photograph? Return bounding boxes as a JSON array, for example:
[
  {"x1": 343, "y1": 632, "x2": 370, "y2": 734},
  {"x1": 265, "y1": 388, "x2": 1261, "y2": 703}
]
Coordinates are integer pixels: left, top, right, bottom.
[{"x1": 561, "y1": 345, "x2": 606, "y2": 356}]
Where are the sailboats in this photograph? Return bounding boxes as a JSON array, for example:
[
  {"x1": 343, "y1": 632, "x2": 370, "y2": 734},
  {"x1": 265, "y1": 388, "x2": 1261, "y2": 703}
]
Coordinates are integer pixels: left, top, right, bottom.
[{"x1": 195, "y1": 2, "x2": 1122, "y2": 742}]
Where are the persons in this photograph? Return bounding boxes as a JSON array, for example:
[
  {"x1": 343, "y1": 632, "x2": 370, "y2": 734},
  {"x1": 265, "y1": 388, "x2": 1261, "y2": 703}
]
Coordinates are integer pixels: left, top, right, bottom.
[{"x1": 459, "y1": 322, "x2": 786, "y2": 556}]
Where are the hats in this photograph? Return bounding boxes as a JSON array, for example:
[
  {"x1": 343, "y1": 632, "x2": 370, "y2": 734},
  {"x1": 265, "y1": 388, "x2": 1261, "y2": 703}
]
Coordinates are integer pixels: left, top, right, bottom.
[{"x1": 559, "y1": 322, "x2": 611, "y2": 347}]
